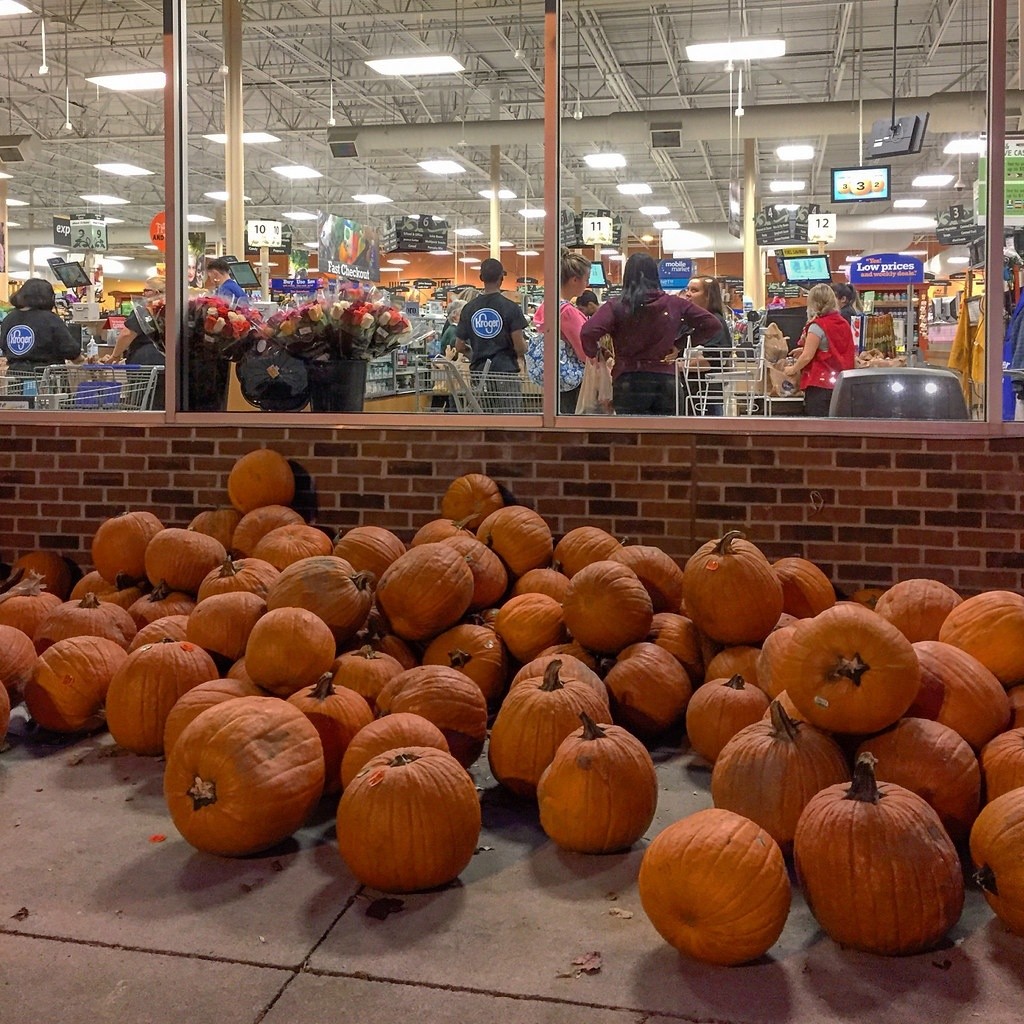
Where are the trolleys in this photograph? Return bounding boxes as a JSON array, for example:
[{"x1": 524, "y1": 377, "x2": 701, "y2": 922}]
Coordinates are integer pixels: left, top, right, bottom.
[
  {"x1": 412, "y1": 352, "x2": 545, "y2": 415},
  {"x1": 0, "y1": 361, "x2": 166, "y2": 413}
]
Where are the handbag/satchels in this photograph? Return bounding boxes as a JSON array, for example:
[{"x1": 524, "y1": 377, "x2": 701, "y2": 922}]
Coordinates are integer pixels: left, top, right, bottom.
[
  {"x1": 527, "y1": 300, "x2": 587, "y2": 392},
  {"x1": 575, "y1": 348, "x2": 613, "y2": 414},
  {"x1": 432, "y1": 345, "x2": 471, "y2": 391}
]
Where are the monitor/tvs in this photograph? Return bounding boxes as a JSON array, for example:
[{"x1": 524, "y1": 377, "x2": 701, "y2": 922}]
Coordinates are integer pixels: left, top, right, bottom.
[
  {"x1": 831, "y1": 164, "x2": 891, "y2": 203},
  {"x1": 864, "y1": 112, "x2": 931, "y2": 160},
  {"x1": 780, "y1": 254, "x2": 832, "y2": 284},
  {"x1": 65, "y1": 323, "x2": 83, "y2": 349}
]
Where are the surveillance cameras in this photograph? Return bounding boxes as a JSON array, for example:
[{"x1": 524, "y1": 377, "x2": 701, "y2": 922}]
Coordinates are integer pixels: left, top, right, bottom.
[{"x1": 954, "y1": 181, "x2": 966, "y2": 192}]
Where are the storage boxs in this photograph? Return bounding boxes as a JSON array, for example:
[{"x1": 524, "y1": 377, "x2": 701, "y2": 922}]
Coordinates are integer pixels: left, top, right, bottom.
[{"x1": 73, "y1": 302, "x2": 100, "y2": 320}]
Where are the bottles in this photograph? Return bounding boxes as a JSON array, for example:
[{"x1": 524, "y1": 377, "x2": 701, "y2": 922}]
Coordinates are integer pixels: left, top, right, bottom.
[
  {"x1": 913, "y1": 330, "x2": 919, "y2": 343},
  {"x1": 860, "y1": 292, "x2": 918, "y2": 323},
  {"x1": 86, "y1": 335, "x2": 98, "y2": 357}
]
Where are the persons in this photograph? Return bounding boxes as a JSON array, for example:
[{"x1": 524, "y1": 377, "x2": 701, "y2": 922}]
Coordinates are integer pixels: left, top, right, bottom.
[
  {"x1": 455, "y1": 258, "x2": 529, "y2": 413},
  {"x1": 785, "y1": 283, "x2": 856, "y2": 418},
  {"x1": 207, "y1": 260, "x2": 247, "y2": 302},
  {"x1": 577, "y1": 290, "x2": 604, "y2": 316},
  {"x1": 102, "y1": 277, "x2": 165, "y2": 412},
  {"x1": 0, "y1": 278, "x2": 85, "y2": 408},
  {"x1": 679, "y1": 276, "x2": 733, "y2": 416},
  {"x1": 580, "y1": 253, "x2": 724, "y2": 414},
  {"x1": 535, "y1": 246, "x2": 591, "y2": 413},
  {"x1": 188, "y1": 260, "x2": 197, "y2": 282},
  {"x1": 833, "y1": 284, "x2": 861, "y2": 322},
  {"x1": 429, "y1": 287, "x2": 479, "y2": 412}
]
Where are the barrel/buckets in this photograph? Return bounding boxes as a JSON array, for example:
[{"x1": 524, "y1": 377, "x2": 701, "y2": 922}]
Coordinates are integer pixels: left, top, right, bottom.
[{"x1": 303, "y1": 359, "x2": 370, "y2": 412}]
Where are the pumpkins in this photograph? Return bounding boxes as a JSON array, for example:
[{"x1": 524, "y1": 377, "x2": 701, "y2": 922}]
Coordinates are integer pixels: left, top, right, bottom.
[{"x1": 0, "y1": 449, "x2": 1024, "y2": 967}]
